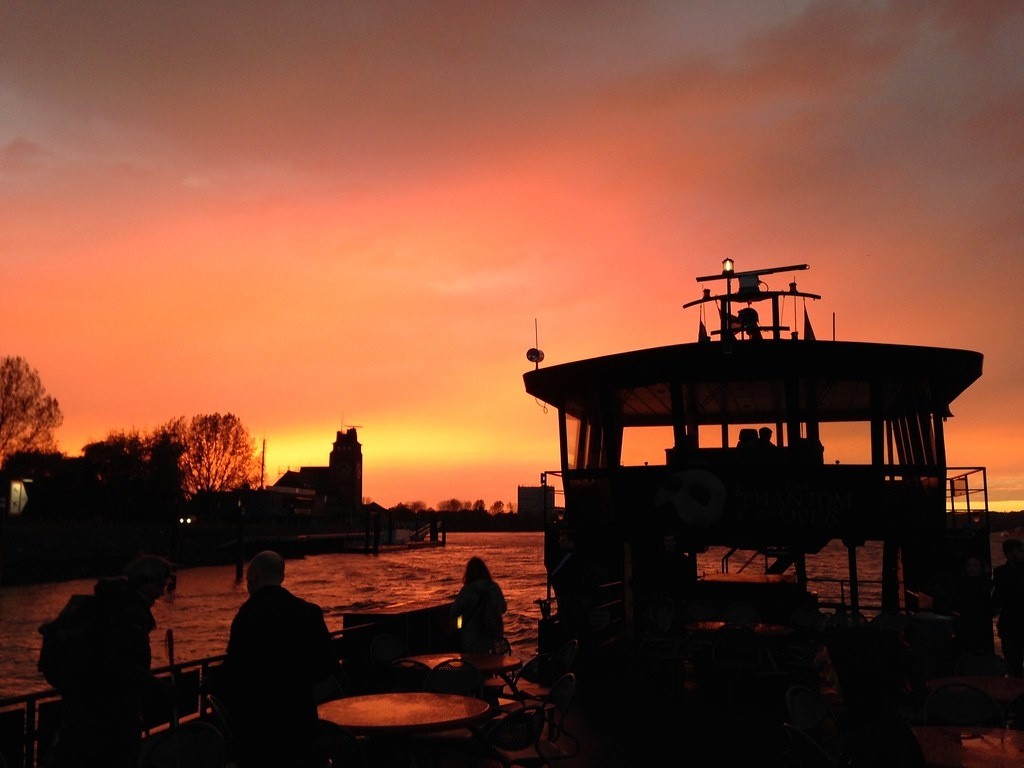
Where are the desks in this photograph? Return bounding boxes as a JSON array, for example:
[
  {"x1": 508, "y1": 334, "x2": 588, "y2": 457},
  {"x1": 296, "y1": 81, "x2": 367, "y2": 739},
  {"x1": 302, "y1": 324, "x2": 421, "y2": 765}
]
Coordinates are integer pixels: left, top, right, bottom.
[
  {"x1": 316, "y1": 694, "x2": 489, "y2": 768},
  {"x1": 920, "y1": 674, "x2": 1024, "y2": 728},
  {"x1": 399, "y1": 653, "x2": 522, "y2": 700},
  {"x1": 697, "y1": 572, "x2": 797, "y2": 609},
  {"x1": 684, "y1": 619, "x2": 794, "y2": 685},
  {"x1": 908, "y1": 724, "x2": 1024, "y2": 768}
]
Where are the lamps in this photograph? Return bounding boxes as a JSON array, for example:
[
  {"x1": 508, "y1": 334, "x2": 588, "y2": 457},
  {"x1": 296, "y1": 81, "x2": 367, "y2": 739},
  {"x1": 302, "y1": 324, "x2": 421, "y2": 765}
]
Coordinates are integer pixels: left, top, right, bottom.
[{"x1": 721, "y1": 257, "x2": 734, "y2": 276}]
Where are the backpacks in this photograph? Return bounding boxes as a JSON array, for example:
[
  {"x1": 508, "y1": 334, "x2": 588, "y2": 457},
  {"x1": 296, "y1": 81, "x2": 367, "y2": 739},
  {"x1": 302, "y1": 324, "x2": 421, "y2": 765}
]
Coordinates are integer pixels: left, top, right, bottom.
[{"x1": 34, "y1": 592, "x2": 118, "y2": 698}]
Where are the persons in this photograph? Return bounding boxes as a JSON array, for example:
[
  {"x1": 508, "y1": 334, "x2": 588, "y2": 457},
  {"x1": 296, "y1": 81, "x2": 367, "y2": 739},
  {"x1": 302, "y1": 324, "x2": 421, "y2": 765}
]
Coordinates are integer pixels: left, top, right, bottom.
[
  {"x1": 455, "y1": 557, "x2": 507, "y2": 656},
  {"x1": 550, "y1": 530, "x2": 582, "y2": 605},
  {"x1": 39, "y1": 556, "x2": 171, "y2": 768},
  {"x1": 754, "y1": 427, "x2": 775, "y2": 450},
  {"x1": 655, "y1": 531, "x2": 679, "y2": 596},
  {"x1": 950, "y1": 539, "x2": 1024, "y2": 678},
  {"x1": 223, "y1": 550, "x2": 328, "y2": 768}
]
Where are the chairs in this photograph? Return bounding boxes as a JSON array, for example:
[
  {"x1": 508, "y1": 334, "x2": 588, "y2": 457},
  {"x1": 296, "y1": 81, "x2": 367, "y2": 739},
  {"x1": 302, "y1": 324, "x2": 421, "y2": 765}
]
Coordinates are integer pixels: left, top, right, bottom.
[
  {"x1": 422, "y1": 659, "x2": 478, "y2": 696},
  {"x1": 141, "y1": 719, "x2": 227, "y2": 768},
  {"x1": 520, "y1": 639, "x2": 578, "y2": 699},
  {"x1": 368, "y1": 633, "x2": 411, "y2": 675},
  {"x1": 388, "y1": 660, "x2": 430, "y2": 692},
  {"x1": 206, "y1": 694, "x2": 233, "y2": 752},
  {"x1": 314, "y1": 718, "x2": 361, "y2": 768},
  {"x1": 481, "y1": 636, "x2": 512, "y2": 686},
  {"x1": 474, "y1": 706, "x2": 545, "y2": 768},
  {"x1": 510, "y1": 673, "x2": 579, "y2": 760},
  {"x1": 627, "y1": 571, "x2": 1024, "y2": 768}
]
